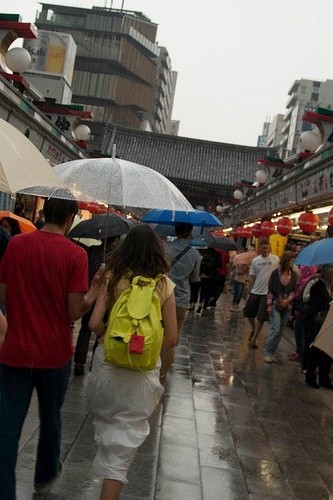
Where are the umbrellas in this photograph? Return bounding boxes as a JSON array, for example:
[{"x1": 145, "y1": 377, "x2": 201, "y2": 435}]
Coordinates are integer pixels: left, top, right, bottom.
[
  {"x1": 0, "y1": 210, "x2": 37, "y2": 234},
  {"x1": 141, "y1": 209, "x2": 240, "y2": 251},
  {"x1": 18, "y1": 144, "x2": 195, "y2": 270},
  {"x1": 293, "y1": 237, "x2": 333, "y2": 266},
  {"x1": 68, "y1": 212, "x2": 136, "y2": 242},
  {"x1": 0, "y1": 117, "x2": 64, "y2": 195}
]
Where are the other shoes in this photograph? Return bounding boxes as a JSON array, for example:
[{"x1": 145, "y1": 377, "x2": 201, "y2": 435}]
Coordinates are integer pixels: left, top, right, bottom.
[
  {"x1": 306, "y1": 383, "x2": 319, "y2": 389},
  {"x1": 74, "y1": 363, "x2": 85, "y2": 376},
  {"x1": 202, "y1": 312, "x2": 208, "y2": 315},
  {"x1": 319, "y1": 382, "x2": 333, "y2": 391},
  {"x1": 248, "y1": 331, "x2": 254, "y2": 341},
  {"x1": 289, "y1": 356, "x2": 299, "y2": 362},
  {"x1": 287, "y1": 353, "x2": 296, "y2": 357},
  {"x1": 271, "y1": 355, "x2": 277, "y2": 362},
  {"x1": 196, "y1": 306, "x2": 202, "y2": 313},
  {"x1": 232, "y1": 302, "x2": 240, "y2": 309},
  {"x1": 188, "y1": 302, "x2": 194, "y2": 310},
  {"x1": 250, "y1": 341, "x2": 258, "y2": 349},
  {"x1": 263, "y1": 355, "x2": 272, "y2": 362},
  {"x1": 34, "y1": 459, "x2": 64, "y2": 493},
  {"x1": 206, "y1": 306, "x2": 214, "y2": 311}
]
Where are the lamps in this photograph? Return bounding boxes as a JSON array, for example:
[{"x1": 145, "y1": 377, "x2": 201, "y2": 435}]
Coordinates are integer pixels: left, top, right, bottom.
[
  {"x1": 299, "y1": 131, "x2": 320, "y2": 152},
  {"x1": 234, "y1": 190, "x2": 243, "y2": 200},
  {"x1": 75, "y1": 124, "x2": 91, "y2": 142},
  {"x1": 254, "y1": 170, "x2": 267, "y2": 184},
  {"x1": 5, "y1": 47, "x2": 30, "y2": 73}
]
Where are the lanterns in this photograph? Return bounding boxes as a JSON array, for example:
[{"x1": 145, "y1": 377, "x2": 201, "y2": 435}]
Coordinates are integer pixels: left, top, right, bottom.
[
  {"x1": 260, "y1": 219, "x2": 275, "y2": 237},
  {"x1": 328, "y1": 208, "x2": 333, "y2": 227},
  {"x1": 215, "y1": 223, "x2": 263, "y2": 239},
  {"x1": 298, "y1": 210, "x2": 319, "y2": 236},
  {"x1": 277, "y1": 217, "x2": 293, "y2": 236}
]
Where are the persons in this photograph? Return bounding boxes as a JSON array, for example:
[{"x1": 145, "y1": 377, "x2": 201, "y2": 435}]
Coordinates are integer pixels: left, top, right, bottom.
[
  {"x1": 189, "y1": 246, "x2": 249, "y2": 313},
  {"x1": 261, "y1": 251, "x2": 296, "y2": 363},
  {"x1": 163, "y1": 222, "x2": 199, "y2": 332},
  {"x1": 73, "y1": 234, "x2": 122, "y2": 374},
  {"x1": 1, "y1": 216, "x2": 21, "y2": 238},
  {"x1": 242, "y1": 239, "x2": 280, "y2": 348},
  {"x1": 0, "y1": 187, "x2": 102, "y2": 500},
  {"x1": 85, "y1": 224, "x2": 178, "y2": 500},
  {"x1": 287, "y1": 262, "x2": 333, "y2": 391}
]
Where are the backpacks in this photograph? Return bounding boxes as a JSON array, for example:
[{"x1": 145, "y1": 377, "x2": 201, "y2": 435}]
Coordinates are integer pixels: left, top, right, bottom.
[{"x1": 103, "y1": 269, "x2": 164, "y2": 372}]
